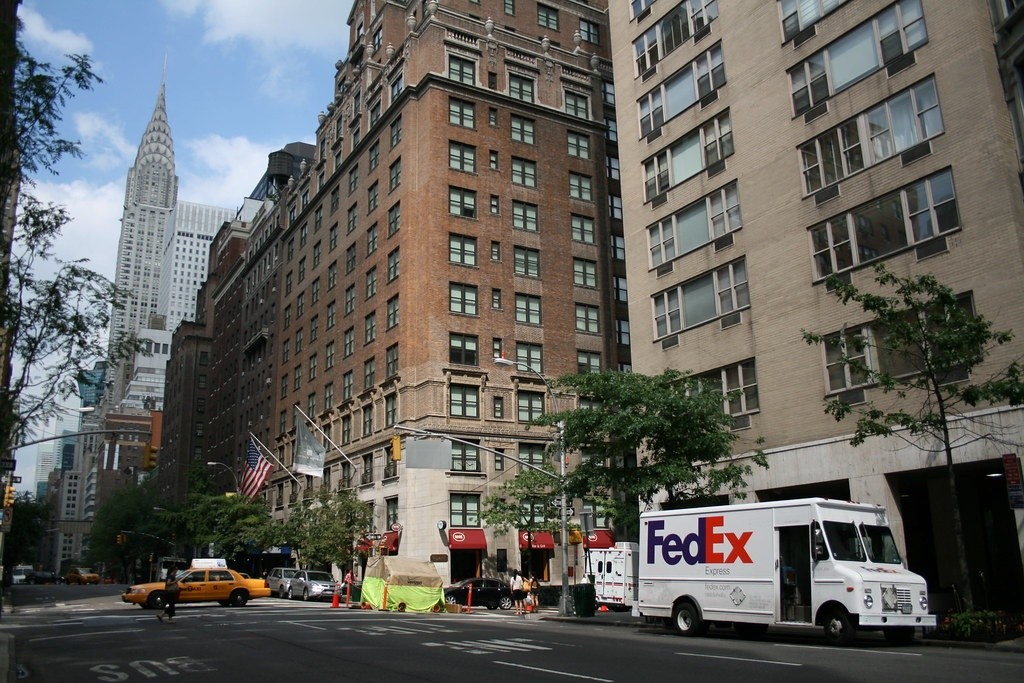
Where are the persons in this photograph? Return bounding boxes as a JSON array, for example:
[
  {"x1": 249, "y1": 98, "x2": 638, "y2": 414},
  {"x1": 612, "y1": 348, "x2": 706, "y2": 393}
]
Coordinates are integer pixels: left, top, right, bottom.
[
  {"x1": 511, "y1": 569, "x2": 539, "y2": 615},
  {"x1": 214, "y1": 575, "x2": 220, "y2": 581},
  {"x1": 345, "y1": 568, "x2": 357, "y2": 586},
  {"x1": 581, "y1": 574, "x2": 586, "y2": 583},
  {"x1": 156, "y1": 567, "x2": 178, "y2": 623}
]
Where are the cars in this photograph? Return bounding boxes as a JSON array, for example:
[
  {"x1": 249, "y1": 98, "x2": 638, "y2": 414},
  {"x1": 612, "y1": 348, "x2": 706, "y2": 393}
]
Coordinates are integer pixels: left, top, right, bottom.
[
  {"x1": 12, "y1": 564, "x2": 114, "y2": 587},
  {"x1": 287, "y1": 570, "x2": 337, "y2": 601},
  {"x1": 443, "y1": 578, "x2": 514, "y2": 610},
  {"x1": 266, "y1": 568, "x2": 298, "y2": 599},
  {"x1": 238, "y1": 573, "x2": 250, "y2": 580},
  {"x1": 121, "y1": 558, "x2": 272, "y2": 611}
]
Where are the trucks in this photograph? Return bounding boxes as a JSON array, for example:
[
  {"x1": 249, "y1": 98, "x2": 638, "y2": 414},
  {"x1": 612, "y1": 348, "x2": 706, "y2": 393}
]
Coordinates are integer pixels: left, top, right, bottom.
[
  {"x1": 640, "y1": 497, "x2": 938, "y2": 646},
  {"x1": 584, "y1": 542, "x2": 640, "y2": 612}
]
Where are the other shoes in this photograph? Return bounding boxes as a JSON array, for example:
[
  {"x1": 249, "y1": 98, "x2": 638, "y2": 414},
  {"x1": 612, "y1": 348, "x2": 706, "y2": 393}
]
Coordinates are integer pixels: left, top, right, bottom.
[
  {"x1": 530, "y1": 611, "x2": 536, "y2": 612},
  {"x1": 157, "y1": 615, "x2": 163, "y2": 623},
  {"x1": 514, "y1": 612, "x2": 519, "y2": 615},
  {"x1": 167, "y1": 619, "x2": 175, "y2": 623},
  {"x1": 519, "y1": 612, "x2": 522, "y2": 615}
]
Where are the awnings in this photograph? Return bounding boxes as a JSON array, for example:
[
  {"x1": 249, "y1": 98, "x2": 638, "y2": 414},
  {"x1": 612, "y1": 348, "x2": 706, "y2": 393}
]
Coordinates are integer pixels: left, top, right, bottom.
[
  {"x1": 356, "y1": 537, "x2": 375, "y2": 551},
  {"x1": 379, "y1": 532, "x2": 398, "y2": 550},
  {"x1": 583, "y1": 529, "x2": 614, "y2": 549},
  {"x1": 449, "y1": 529, "x2": 487, "y2": 549},
  {"x1": 518, "y1": 529, "x2": 554, "y2": 548}
]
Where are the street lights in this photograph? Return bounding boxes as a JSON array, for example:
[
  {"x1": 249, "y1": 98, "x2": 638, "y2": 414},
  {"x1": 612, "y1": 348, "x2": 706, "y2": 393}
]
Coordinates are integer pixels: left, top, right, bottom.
[
  {"x1": 493, "y1": 356, "x2": 573, "y2": 616},
  {"x1": 206, "y1": 461, "x2": 239, "y2": 494}
]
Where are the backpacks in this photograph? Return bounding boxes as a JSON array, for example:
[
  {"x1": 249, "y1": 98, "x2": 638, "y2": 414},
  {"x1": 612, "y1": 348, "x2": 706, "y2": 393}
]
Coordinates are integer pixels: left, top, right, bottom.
[{"x1": 531, "y1": 578, "x2": 540, "y2": 595}]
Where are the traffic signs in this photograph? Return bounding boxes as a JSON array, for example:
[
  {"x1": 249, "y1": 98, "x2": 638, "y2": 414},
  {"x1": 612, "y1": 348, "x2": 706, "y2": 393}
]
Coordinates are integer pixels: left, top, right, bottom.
[
  {"x1": 559, "y1": 508, "x2": 576, "y2": 517},
  {"x1": 0, "y1": 476, "x2": 21, "y2": 483}
]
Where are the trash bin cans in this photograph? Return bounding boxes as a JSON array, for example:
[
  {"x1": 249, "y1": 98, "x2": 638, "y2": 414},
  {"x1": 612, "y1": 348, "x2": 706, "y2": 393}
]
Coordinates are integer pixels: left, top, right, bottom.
[
  {"x1": 351, "y1": 581, "x2": 362, "y2": 602},
  {"x1": 573, "y1": 584, "x2": 595, "y2": 617}
]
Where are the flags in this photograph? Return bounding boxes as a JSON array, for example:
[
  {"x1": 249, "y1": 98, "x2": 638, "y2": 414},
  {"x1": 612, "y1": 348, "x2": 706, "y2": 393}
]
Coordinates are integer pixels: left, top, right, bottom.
[
  {"x1": 240, "y1": 437, "x2": 274, "y2": 504},
  {"x1": 293, "y1": 411, "x2": 325, "y2": 476}
]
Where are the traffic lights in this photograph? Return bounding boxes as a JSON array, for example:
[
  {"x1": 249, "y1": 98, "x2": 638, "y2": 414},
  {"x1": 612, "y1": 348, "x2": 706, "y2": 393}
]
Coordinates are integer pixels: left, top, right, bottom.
[{"x1": 117, "y1": 534, "x2": 123, "y2": 544}]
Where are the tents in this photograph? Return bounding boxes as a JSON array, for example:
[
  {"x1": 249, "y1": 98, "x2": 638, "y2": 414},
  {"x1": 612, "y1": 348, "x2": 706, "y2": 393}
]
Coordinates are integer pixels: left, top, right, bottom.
[{"x1": 361, "y1": 555, "x2": 446, "y2": 612}]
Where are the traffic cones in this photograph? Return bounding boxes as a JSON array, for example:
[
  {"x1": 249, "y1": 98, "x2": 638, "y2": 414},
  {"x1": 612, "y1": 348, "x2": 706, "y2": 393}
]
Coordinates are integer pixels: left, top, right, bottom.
[{"x1": 330, "y1": 583, "x2": 342, "y2": 608}]
